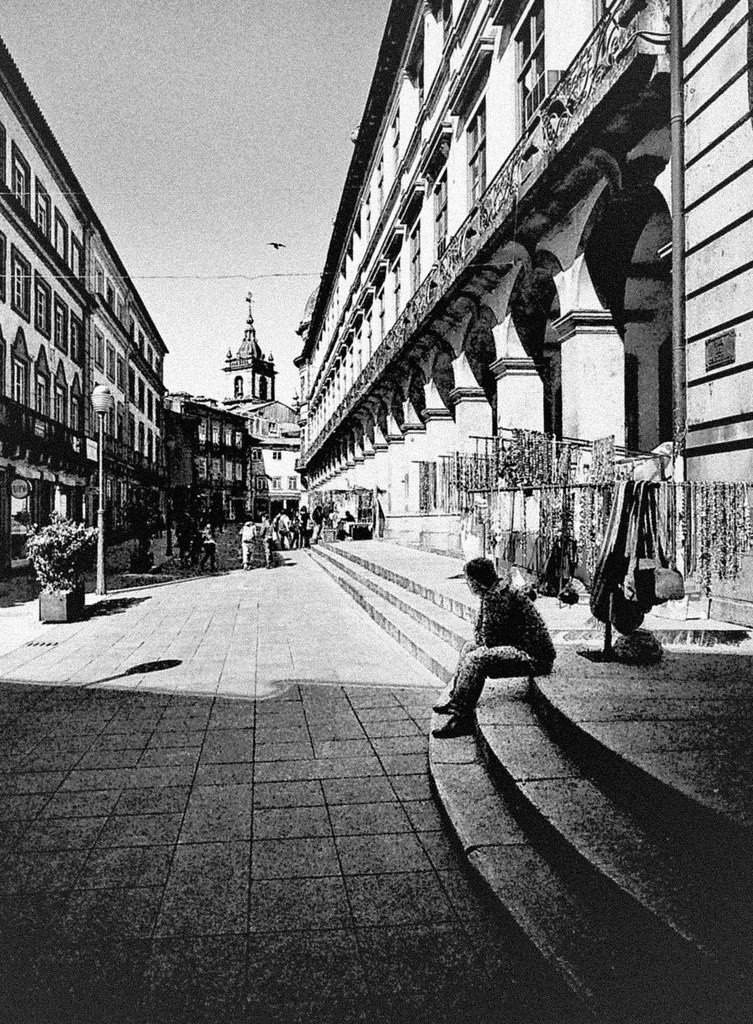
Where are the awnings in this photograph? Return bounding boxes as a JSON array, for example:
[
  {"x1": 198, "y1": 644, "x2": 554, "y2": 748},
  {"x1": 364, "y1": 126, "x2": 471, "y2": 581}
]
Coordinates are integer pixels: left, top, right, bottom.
[
  {"x1": 312, "y1": 472, "x2": 390, "y2": 499},
  {"x1": 14, "y1": 466, "x2": 87, "y2": 487}
]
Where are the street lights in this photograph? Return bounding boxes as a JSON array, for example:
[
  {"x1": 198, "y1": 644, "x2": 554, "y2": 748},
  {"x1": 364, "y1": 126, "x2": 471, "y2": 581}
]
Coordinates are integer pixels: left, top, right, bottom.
[{"x1": 91, "y1": 383, "x2": 113, "y2": 594}]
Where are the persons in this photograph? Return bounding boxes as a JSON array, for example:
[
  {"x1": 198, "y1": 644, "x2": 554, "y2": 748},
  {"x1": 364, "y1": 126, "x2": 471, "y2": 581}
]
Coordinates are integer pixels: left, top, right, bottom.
[
  {"x1": 174, "y1": 503, "x2": 358, "y2": 576},
  {"x1": 433, "y1": 557, "x2": 557, "y2": 742}
]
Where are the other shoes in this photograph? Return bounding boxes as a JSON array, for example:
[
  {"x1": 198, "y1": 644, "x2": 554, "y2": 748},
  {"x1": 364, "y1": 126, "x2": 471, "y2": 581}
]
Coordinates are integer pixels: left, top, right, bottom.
[
  {"x1": 432, "y1": 715, "x2": 473, "y2": 738},
  {"x1": 433, "y1": 699, "x2": 452, "y2": 715}
]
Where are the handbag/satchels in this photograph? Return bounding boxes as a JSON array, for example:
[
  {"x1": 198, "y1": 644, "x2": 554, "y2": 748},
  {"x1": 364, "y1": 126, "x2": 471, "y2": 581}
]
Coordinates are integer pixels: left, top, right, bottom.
[{"x1": 589, "y1": 481, "x2": 685, "y2": 636}]
